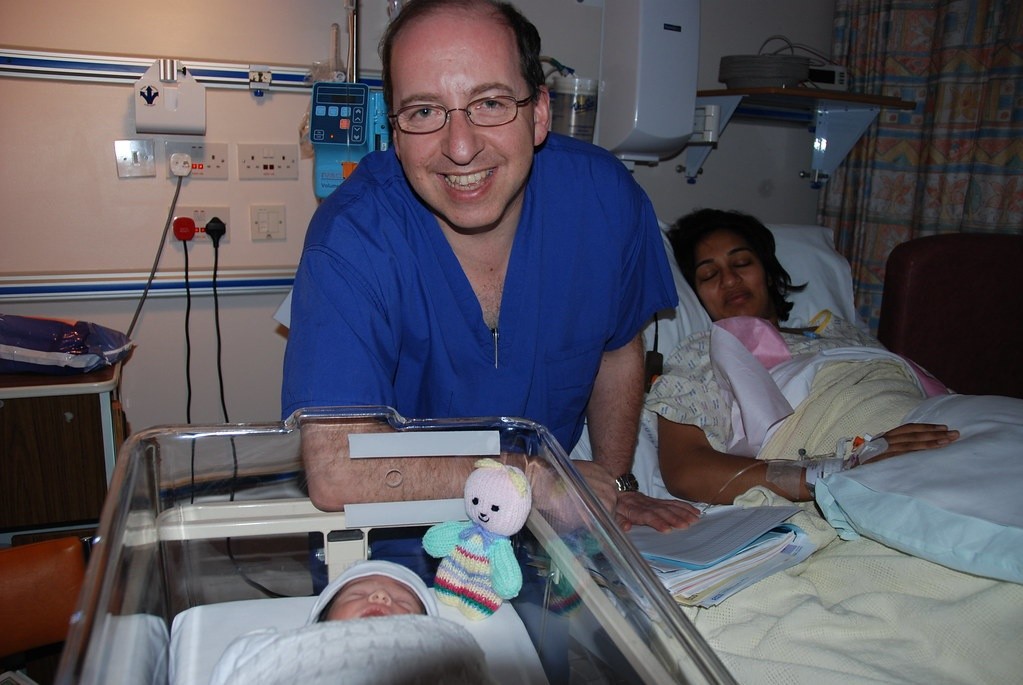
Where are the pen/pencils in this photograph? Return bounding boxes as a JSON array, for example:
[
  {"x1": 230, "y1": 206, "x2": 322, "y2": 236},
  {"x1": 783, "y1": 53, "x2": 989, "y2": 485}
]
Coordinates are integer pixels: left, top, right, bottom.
[{"x1": 492, "y1": 327, "x2": 499, "y2": 368}]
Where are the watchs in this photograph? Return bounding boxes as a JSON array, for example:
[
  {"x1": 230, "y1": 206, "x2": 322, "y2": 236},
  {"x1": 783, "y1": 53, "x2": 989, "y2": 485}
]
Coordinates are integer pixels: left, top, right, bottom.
[{"x1": 615, "y1": 473, "x2": 639, "y2": 492}]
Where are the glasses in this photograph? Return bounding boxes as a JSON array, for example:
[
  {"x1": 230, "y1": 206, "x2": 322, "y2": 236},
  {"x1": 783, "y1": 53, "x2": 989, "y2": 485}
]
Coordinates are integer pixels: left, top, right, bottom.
[{"x1": 387, "y1": 94, "x2": 532, "y2": 134}]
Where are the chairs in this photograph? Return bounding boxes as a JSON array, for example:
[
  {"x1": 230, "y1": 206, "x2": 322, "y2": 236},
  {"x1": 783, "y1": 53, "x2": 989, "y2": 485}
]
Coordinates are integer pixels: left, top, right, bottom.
[{"x1": 876, "y1": 233, "x2": 1023, "y2": 403}]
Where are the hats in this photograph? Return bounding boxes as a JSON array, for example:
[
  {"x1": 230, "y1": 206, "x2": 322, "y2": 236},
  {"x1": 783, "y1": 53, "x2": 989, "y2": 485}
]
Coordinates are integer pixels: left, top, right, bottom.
[{"x1": 307, "y1": 559, "x2": 438, "y2": 622}]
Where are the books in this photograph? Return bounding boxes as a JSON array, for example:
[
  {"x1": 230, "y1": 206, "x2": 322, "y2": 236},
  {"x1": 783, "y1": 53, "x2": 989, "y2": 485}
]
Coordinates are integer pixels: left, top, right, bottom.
[{"x1": 524, "y1": 527, "x2": 796, "y2": 598}]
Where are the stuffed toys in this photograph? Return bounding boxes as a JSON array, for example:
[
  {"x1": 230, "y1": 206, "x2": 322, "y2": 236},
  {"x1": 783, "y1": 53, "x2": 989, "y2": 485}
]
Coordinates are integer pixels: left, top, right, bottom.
[{"x1": 422, "y1": 457, "x2": 533, "y2": 618}]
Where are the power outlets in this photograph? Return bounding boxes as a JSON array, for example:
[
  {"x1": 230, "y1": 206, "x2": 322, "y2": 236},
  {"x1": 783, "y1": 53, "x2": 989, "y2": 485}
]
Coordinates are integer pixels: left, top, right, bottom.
[
  {"x1": 248, "y1": 202, "x2": 290, "y2": 239},
  {"x1": 236, "y1": 141, "x2": 299, "y2": 179},
  {"x1": 170, "y1": 138, "x2": 227, "y2": 181},
  {"x1": 171, "y1": 203, "x2": 230, "y2": 244}
]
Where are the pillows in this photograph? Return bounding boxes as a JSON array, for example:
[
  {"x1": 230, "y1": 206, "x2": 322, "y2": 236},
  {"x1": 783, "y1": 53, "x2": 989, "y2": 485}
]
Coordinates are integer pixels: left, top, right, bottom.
[{"x1": 640, "y1": 226, "x2": 857, "y2": 363}]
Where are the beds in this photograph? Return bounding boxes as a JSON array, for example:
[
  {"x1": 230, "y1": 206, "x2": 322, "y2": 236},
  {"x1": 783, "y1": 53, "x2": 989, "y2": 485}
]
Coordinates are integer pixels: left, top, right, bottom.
[{"x1": 570, "y1": 220, "x2": 1023, "y2": 684}]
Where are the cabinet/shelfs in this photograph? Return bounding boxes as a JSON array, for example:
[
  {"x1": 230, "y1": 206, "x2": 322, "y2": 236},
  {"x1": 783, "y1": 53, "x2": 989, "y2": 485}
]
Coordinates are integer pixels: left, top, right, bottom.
[{"x1": 0, "y1": 363, "x2": 125, "y2": 555}]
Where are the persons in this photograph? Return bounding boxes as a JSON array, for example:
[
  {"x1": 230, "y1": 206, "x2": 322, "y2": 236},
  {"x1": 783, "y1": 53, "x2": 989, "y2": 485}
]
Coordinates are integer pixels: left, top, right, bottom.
[
  {"x1": 282, "y1": 0, "x2": 701, "y2": 532},
  {"x1": 664, "y1": 208, "x2": 960, "y2": 506},
  {"x1": 210, "y1": 560, "x2": 489, "y2": 685}
]
